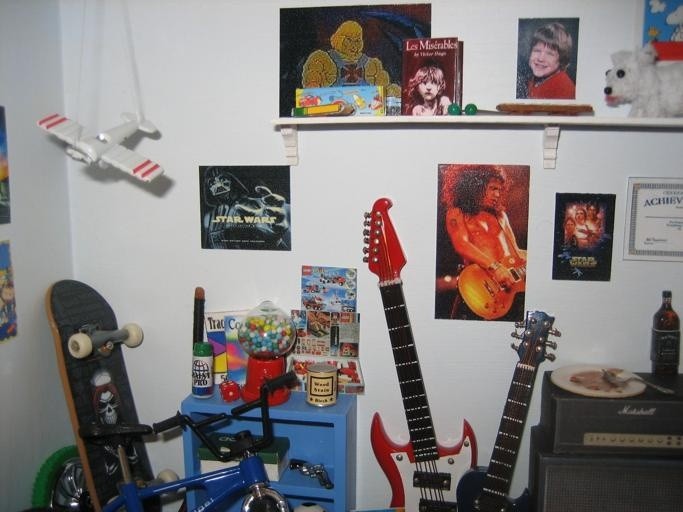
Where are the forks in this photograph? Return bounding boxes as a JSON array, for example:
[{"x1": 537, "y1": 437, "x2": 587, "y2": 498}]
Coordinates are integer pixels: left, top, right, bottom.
[{"x1": 602, "y1": 367, "x2": 673, "y2": 397}]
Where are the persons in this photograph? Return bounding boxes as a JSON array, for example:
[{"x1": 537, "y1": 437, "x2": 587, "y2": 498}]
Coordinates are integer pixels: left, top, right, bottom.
[
  {"x1": 563, "y1": 200, "x2": 602, "y2": 250},
  {"x1": 442, "y1": 164, "x2": 526, "y2": 319},
  {"x1": 528, "y1": 22, "x2": 575, "y2": 99},
  {"x1": 409, "y1": 66, "x2": 452, "y2": 116},
  {"x1": 202, "y1": 164, "x2": 291, "y2": 252}
]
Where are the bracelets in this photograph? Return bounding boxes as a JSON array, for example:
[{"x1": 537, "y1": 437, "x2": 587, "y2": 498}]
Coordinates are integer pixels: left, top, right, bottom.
[{"x1": 488, "y1": 261, "x2": 501, "y2": 272}]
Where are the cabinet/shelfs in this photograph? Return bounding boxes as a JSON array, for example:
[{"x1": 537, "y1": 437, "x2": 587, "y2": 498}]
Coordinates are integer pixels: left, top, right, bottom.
[{"x1": 181, "y1": 389, "x2": 355, "y2": 512}]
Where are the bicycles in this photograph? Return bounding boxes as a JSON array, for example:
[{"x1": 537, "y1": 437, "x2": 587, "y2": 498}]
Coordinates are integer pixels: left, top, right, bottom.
[{"x1": 78, "y1": 370, "x2": 296, "y2": 512}]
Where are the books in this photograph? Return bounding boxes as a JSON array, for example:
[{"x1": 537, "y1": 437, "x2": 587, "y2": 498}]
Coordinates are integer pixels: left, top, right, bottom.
[
  {"x1": 203, "y1": 310, "x2": 250, "y2": 389},
  {"x1": 402, "y1": 37, "x2": 464, "y2": 115}
]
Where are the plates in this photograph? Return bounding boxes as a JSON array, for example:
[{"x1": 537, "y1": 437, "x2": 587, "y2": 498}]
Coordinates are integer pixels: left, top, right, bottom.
[{"x1": 550, "y1": 365, "x2": 646, "y2": 398}]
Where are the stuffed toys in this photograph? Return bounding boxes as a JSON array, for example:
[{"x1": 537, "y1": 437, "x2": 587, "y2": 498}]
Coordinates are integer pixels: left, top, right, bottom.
[{"x1": 604, "y1": 42, "x2": 683, "y2": 118}]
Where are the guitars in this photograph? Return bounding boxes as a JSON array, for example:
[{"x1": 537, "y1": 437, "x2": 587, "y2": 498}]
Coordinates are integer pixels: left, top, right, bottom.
[
  {"x1": 455, "y1": 311, "x2": 562, "y2": 511},
  {"x1": 362, "y1": 198, "x2": 478, "y2": 512},
  {"x1": 456, "y1": 250, "x2": 526, "y2": 319}
]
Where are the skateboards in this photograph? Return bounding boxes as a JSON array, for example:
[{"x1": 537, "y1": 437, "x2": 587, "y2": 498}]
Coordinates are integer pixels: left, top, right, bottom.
[{"x1": 45, "y1": 280, "x2": 163, "y2": 511}]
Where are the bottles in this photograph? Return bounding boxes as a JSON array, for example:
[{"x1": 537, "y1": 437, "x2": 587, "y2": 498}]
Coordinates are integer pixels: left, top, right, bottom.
[
  {"x1": 649, "y1": 292, "x2": 680, "y2": 386},
  {"x1": 191, "y1": 341, "x2": 215, "y2": 399}
]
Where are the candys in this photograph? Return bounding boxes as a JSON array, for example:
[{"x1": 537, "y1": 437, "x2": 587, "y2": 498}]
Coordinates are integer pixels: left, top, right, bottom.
[{"x1": 238, "y1": 319, "x2": 293, "y2": 356}]
